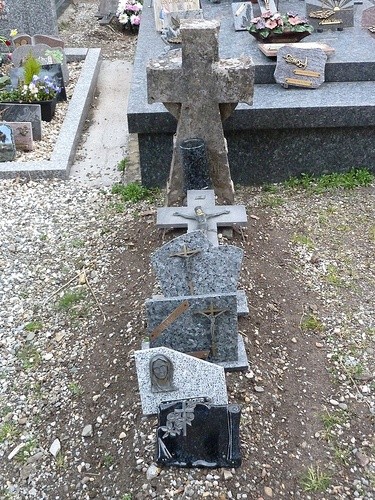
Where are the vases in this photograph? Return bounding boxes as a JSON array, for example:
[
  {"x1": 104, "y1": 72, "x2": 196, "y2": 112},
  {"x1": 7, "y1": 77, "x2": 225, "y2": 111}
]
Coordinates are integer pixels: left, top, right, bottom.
[
  {"x1": 249, "y1": 30, "x2": 312, "y2": 43},
  {"x1": 0, "y1": 94, "x2": 57, "y2": 122}
]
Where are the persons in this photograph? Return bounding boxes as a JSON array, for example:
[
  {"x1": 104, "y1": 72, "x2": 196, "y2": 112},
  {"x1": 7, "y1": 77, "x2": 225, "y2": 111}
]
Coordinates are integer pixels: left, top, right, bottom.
[
  {"x1": 173, "y1": 206, "x2": 230, "y2": 231},
  {"x1": 150, "y1": 354, "x2": 176, "y2": 393}
]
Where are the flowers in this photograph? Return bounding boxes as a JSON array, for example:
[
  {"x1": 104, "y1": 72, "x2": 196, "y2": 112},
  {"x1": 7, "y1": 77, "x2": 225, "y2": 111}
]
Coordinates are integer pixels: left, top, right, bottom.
[
  {"x1": 248, "y1": 10, "x2": 315, "y2": 39},
  {"x1": 0, "y1": 29, "x2": 18, "y2": 53},
  {"x1": 0, "y1": 74, "x2": 61, "y2": 103},
  {"x1": 116, "y1": 0, "x2": 143, "y2": 33}
]
